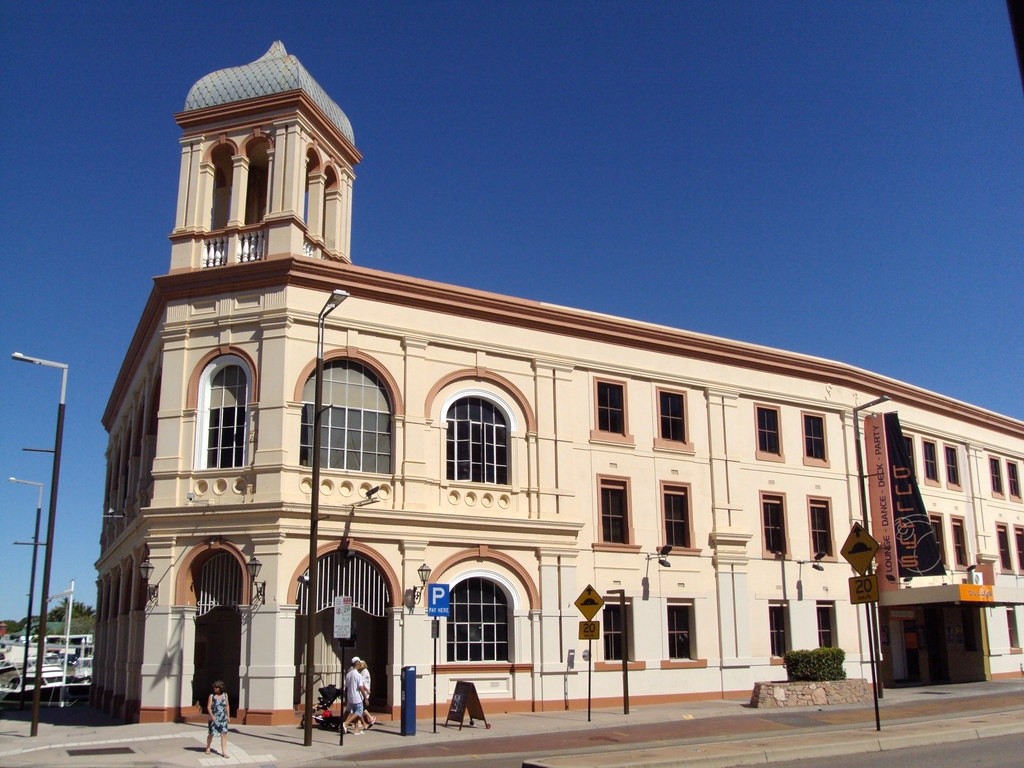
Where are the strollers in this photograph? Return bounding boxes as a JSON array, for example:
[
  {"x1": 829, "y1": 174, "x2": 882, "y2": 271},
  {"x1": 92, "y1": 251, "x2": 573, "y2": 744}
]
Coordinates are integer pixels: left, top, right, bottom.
[{"x1": 300, "y1": 682, "x2": 347, "y2": 735}]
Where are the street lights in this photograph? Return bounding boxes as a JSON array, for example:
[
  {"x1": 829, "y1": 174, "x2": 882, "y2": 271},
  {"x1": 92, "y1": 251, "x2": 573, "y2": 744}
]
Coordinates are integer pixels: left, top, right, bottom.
[
  {"x1": 10, "y1": 352, "x2": 69, "y2": 741},
  {"x1": 302, "y1": 287, "x2": 351, "y2": 748},
  {"x1": 8, "y1": 477, "x2": 44, "y2": 712}
]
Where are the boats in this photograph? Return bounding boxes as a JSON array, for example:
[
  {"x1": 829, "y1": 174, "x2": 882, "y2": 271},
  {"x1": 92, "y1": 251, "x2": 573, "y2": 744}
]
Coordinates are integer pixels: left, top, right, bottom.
[{"x1": 0, "y1": 633, "x2": 96, "y2": 702}]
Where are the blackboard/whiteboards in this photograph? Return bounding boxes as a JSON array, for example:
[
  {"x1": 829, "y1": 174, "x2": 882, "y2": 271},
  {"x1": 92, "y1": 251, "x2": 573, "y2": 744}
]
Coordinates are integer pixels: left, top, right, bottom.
[{"x1": 447, "y1": 681, "x2": 472, "y2": 722}]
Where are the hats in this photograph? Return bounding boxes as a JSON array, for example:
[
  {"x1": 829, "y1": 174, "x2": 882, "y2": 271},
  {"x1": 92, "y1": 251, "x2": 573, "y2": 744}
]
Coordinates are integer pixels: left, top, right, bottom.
[{"x1": 351, "y1": 656, "x2": 360, "y2": 663}]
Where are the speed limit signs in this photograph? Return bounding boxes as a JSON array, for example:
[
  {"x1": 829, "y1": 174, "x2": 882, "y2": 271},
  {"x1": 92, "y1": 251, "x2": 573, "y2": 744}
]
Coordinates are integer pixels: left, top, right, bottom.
[
  {"x1": 580, "y1": 620, "x2": 599, "y2": 641},
  {"x1": 847, "y1": 576, "x2": 878, "y2": 604}
]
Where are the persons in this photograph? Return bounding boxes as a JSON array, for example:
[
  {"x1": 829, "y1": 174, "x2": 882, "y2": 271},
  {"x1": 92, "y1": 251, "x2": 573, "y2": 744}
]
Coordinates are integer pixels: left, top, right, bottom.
[
  {"x1": 205, "y1": 679, "x2": 231, "y2": 759},
  {"x1": 342, "y1": 656, "x2": 377, "y2": 735}
]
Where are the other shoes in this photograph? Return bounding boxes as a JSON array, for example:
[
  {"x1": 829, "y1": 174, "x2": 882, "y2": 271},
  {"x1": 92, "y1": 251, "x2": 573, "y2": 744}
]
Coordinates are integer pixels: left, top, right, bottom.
[
  {"x1": 370, "y1": 716, "x2": 376, "y2": 724},
  {"x1": 206, "y1": 748, "x2": 211, "y2": 754},
  {"x1": 343, "y1": 722, "x2": 347, "y2": 734},
  {"x1": 363, "y1": 724, "x2": 372, "y2": 730},
  {"x1": 353, "y1": 723, "x2": 363, "y2": 727},
  {"x1": 353, "y1": 731, "x2": 364, "y2": 736},
  {"x1": 222, "y1": 753, "x2": 230, "y2": 758}
]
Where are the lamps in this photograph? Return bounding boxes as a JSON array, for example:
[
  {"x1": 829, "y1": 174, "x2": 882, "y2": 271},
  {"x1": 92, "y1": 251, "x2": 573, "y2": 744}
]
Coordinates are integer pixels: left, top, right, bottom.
[
  {"x1": 798, "y1": 552, "x2": 826, "y2": 571},
  {"x1": 246, "y1": 558, "x2": 266, "y2": 606},
  {"x1": 411, "y1": 563, "x2": 432, "y2": 604},
  {"x1": 140, "y1": 559, "x2": 160, "y2": 606},
  {"x1": 644, "y1": 545, "x2": 673, "y2": 568}
]
